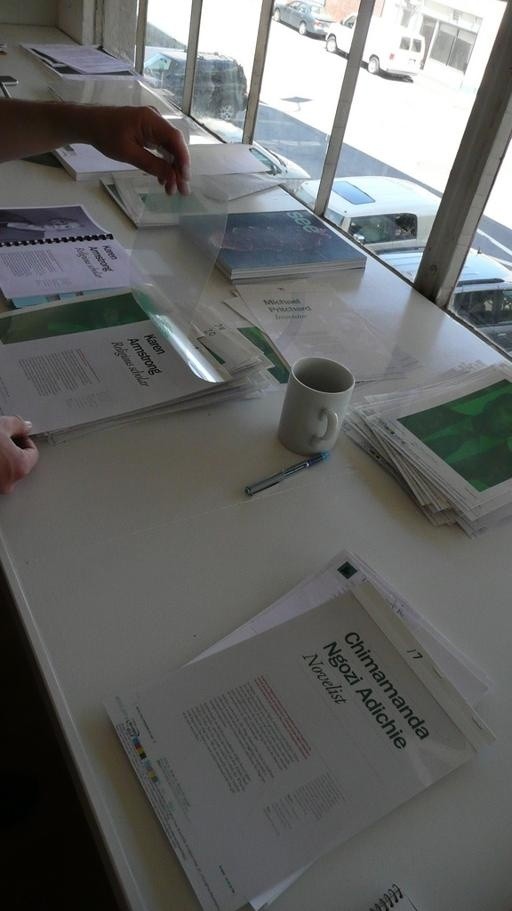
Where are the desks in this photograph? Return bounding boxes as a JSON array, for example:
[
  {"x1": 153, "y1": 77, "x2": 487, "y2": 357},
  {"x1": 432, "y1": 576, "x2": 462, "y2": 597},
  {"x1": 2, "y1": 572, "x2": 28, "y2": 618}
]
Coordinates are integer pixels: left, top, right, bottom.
[{"x1": 0, "y1": 23, "x2": 512, "y2": 911}]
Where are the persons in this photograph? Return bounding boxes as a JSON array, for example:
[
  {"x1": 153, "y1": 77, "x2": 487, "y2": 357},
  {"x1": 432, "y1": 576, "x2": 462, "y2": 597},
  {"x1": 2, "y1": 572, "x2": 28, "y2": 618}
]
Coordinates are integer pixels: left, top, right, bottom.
[
  {"x1": 1, "y1": 93, "x2": 192, "y2": 199},
  {"x1": 0, "y1": 412, "x2": 42, "y2": 494}
]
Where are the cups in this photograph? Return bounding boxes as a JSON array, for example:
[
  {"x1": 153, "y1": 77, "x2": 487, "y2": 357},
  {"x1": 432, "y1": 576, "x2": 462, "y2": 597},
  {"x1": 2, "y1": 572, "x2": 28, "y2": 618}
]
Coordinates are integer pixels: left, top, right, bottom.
[{"x1": 277, "y1": 356, "x2": 356, "y2": 460}]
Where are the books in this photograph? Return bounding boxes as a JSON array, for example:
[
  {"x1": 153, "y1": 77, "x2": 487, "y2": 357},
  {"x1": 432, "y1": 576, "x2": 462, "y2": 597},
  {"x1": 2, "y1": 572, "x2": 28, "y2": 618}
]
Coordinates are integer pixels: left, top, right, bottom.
[{"x1": 1, "y1": 144, "x2": 368, "y2": 307}]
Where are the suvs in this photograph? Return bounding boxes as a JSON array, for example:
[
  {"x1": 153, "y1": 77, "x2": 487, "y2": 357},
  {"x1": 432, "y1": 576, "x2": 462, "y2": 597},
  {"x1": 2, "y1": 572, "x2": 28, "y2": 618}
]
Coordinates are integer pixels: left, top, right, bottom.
[
  {"x1": 140, "y1": 50, "x2": 248, "y2": 123},
  {"x1": 379, "y1": 246, "x2": 512, "y2": 351}
]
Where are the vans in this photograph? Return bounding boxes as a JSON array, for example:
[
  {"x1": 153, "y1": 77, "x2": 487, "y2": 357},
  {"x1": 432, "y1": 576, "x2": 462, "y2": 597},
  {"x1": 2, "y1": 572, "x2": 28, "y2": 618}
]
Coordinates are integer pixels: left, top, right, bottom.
[
  {"x1": 201, "y1": 118, "x2": 311, "y2": 181},
  {"x1": 325, "y1": 11, "x2": 426, "y2": 81}
]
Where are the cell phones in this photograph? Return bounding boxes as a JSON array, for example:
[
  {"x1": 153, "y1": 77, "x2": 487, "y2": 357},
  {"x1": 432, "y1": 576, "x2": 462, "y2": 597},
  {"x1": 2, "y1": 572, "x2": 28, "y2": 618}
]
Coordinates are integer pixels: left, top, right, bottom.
[{"x1": 0, "y1": 75, "x2": 18, "y2": 84}]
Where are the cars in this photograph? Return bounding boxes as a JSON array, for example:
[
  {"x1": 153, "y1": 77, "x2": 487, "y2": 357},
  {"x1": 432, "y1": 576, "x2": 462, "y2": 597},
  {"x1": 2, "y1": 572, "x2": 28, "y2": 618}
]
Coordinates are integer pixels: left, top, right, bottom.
[{"x1": 272, "y1": 0, "x2": 336, "y2": 38}]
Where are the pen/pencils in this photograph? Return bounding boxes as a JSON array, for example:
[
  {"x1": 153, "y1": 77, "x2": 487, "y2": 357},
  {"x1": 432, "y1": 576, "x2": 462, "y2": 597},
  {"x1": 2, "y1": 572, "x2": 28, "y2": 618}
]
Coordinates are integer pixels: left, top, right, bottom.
[{"x1": 244, "y1": 451, "x2": 329, "y2": 498}]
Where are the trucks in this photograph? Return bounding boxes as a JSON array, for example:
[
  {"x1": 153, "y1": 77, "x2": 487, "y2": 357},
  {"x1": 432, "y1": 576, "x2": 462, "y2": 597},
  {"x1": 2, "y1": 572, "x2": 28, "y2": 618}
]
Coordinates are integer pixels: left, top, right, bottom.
[{"x1": 293, "y1": 177, "x2": 450, "y2": 254}]
